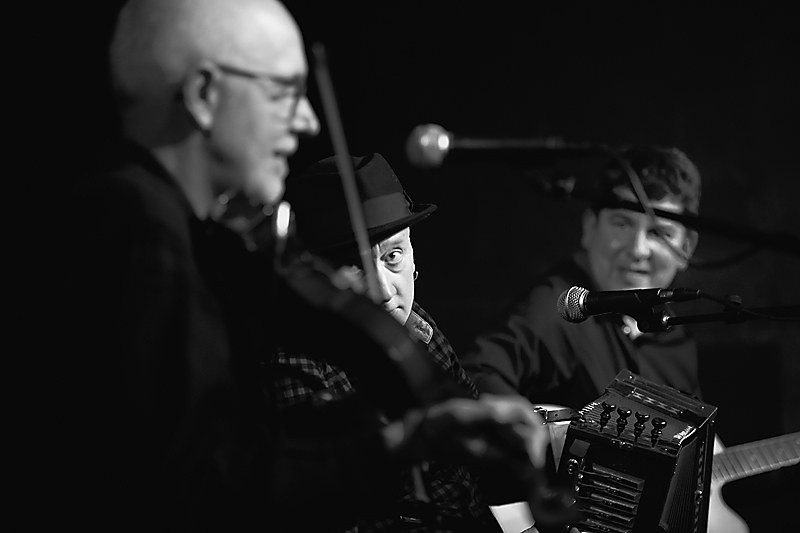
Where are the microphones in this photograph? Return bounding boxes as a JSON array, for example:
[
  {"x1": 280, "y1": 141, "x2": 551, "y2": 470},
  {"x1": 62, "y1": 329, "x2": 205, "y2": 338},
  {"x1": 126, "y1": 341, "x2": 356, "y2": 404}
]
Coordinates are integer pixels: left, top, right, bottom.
[
  {"x1": 403, "y1": 123, "x2": 612, "y2": 170},
  {"x1": 557, "y1": 285, "x2": 703, "y2": 323}
]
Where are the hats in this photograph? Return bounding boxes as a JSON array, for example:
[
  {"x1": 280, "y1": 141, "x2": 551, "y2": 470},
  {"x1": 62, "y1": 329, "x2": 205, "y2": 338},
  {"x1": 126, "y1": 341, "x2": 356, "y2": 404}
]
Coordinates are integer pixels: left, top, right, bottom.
[{"x1": 289, "y1": 153, "x2": 439, "y2": 263}]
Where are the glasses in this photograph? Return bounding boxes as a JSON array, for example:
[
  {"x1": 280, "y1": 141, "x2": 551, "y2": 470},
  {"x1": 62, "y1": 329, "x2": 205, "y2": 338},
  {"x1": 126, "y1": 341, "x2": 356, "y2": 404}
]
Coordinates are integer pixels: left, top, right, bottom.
[{"x1": 220, "y1": 63, "x2": 307, "y2": 100}]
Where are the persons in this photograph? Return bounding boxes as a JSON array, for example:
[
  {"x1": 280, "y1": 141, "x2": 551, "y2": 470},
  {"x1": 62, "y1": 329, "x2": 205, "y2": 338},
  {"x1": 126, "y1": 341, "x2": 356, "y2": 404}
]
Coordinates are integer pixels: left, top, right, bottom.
[
  {"x1": 0, "y1": 0, "x2": 550, "y2": 533},
  {"x1": 460, "y1": 147, "x2": 704, "y2": 533},
  {"x1": 287, "y1": 152, "x2": 483, "y2": 533}
]
